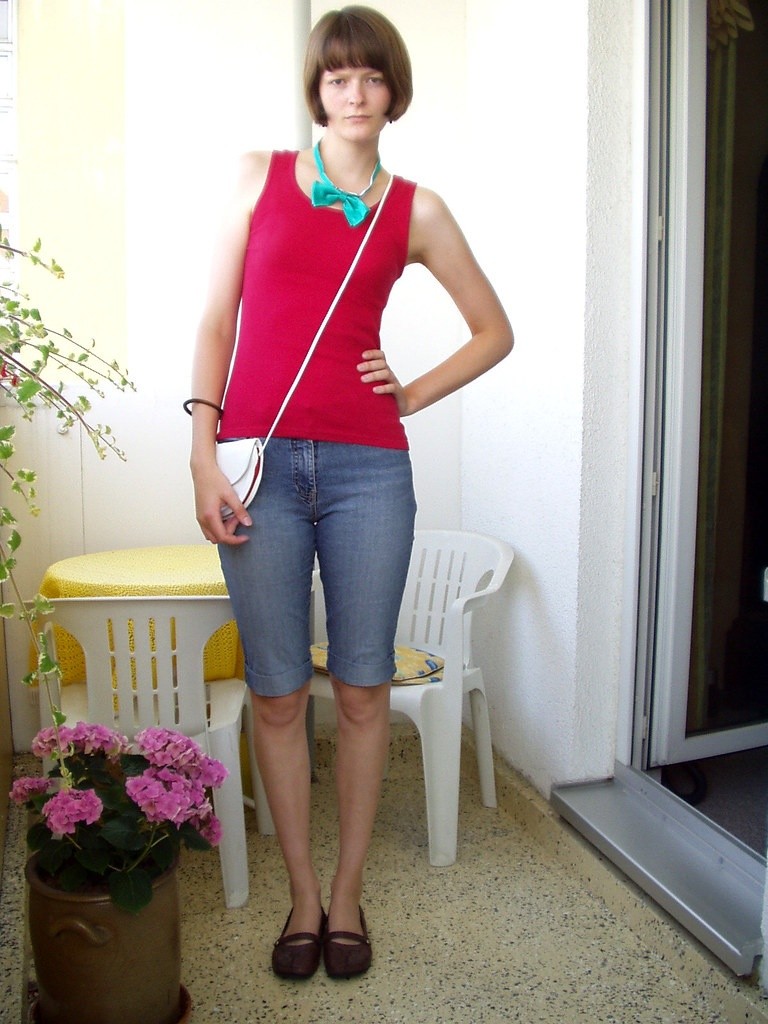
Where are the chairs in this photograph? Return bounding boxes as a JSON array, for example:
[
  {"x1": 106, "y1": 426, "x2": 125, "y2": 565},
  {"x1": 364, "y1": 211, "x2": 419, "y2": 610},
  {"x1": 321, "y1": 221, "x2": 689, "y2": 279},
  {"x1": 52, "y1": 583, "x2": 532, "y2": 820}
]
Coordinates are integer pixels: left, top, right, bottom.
[
  {"x1": 21, "y1": 593, "x2": 278, "y2": 908},
  {"x1": 301, "y1": 530, "x2": 514, "y2": 868}
]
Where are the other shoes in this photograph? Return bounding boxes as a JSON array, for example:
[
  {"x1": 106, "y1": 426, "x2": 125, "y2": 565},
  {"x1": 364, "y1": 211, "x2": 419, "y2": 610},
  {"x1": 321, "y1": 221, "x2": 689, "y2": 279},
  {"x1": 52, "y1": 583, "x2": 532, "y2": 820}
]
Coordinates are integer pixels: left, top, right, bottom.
[
  {"x1": 271, "y1": 905, "x2": 326, "y2": 980},
  {"x1": 323, "y1": 901, "x2": 372, "y2": 979}
]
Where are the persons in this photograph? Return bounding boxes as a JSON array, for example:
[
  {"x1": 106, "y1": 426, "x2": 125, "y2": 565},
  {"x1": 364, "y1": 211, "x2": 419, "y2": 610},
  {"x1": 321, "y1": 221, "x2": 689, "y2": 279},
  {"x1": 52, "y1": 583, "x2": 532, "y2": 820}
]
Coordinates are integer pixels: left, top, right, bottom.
[{"x1": 181, "y1": 3, "x2": 514, "y2": 985}]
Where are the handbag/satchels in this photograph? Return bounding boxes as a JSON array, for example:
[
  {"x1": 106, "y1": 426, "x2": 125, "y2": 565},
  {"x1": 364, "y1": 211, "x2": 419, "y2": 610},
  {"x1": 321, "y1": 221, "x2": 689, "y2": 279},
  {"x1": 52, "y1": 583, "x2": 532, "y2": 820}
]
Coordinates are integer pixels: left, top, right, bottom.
[{"x1": 215, "y1": 438, "x2": 264, "y2": 520}]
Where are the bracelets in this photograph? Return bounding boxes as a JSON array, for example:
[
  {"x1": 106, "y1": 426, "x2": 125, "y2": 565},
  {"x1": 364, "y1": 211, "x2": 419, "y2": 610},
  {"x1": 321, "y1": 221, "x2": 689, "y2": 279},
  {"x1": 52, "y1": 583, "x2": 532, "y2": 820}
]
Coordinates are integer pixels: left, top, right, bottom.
[{"x1": 183, "y1": 399, "x2": 224, "y2": 420}]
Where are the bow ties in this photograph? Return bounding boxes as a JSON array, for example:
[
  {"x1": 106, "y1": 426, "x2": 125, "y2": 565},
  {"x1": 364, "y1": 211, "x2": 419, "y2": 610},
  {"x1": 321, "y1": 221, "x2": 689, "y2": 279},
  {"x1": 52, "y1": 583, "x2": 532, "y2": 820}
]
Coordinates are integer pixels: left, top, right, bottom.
[{"x1": 311, "y1": 137, "x2": 381, "y2": 226}]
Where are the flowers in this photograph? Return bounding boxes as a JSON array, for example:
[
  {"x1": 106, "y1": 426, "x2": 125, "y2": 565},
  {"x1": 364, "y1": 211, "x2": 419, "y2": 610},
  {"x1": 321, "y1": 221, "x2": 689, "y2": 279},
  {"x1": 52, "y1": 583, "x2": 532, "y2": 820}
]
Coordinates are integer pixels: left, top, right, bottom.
[{"x1": 8, "y1": 729, "x2": 228, "y2": 912}]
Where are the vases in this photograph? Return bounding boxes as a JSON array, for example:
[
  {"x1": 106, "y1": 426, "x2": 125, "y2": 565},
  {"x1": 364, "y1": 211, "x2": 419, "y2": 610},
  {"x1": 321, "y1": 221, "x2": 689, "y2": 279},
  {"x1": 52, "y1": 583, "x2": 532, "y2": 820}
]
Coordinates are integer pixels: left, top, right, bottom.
[{"x1": 28, "y1": 854, "x2": 193, "y2": 1024}]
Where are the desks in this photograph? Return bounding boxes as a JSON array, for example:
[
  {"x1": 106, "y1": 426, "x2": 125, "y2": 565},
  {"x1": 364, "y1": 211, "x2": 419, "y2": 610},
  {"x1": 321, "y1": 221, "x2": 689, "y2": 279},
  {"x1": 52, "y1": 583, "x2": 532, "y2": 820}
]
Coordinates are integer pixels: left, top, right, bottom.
[{"x1": 32, "y1": 545, "x2": 277, "y2": 778}]
